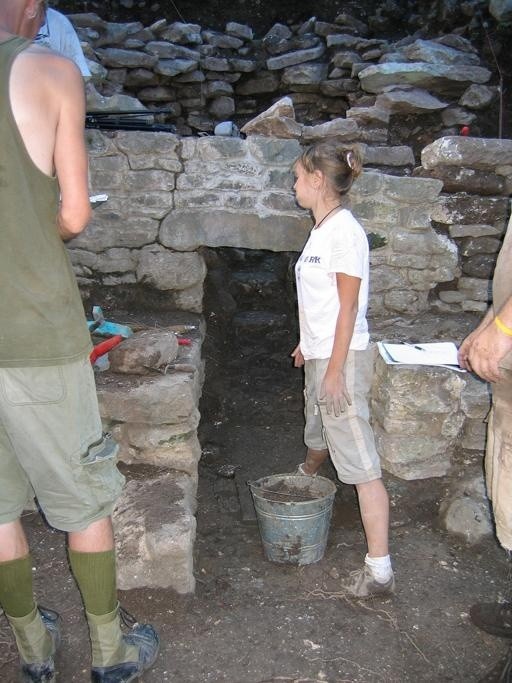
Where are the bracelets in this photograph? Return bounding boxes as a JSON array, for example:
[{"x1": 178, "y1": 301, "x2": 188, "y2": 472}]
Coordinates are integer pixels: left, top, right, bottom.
[{"x1": 492, "y1": 316, "x2": 511, "y2": 338}]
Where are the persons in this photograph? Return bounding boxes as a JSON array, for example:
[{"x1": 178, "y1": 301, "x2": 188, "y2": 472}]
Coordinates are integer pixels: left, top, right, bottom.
[
  {"x1": 456, "y1": 215, "x2": 512, "y2": 638},
  {"x1": 0, "y1": 0, "x2": 159, "y2": 683},
  {"x1": 289, "y1": 144, "x2": 396, "y2": 602},
  {"x1": 29, "y1": 0, "x2": 91, "y2": 79}
]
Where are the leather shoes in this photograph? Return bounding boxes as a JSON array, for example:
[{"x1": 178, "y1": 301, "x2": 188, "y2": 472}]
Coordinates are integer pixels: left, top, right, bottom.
[
  {"x1": 17, "y1": 601, "x2": 60, "y2": 683},
  {"x1": 90, "y1": 620, "x2": 162, "y2": 683}
]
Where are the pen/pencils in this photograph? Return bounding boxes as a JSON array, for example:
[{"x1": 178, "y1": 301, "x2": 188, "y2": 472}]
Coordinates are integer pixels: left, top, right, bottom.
[{"x1": 400, "y1": 340, "x2": 426, "y2": 352}]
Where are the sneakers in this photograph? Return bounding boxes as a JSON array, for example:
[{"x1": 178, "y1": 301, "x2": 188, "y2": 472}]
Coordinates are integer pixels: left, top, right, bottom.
[
  {"x1": 468, "y1": 600, "x2": 512, "y2": 642},
  {"x1": 322, "y1": 565, "x2": 398, "y2": 606}
]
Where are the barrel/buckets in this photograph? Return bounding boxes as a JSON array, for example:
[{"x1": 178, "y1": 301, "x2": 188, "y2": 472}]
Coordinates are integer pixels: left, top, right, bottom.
[{"x1": 246, "y1": 474, "x2": 338, "y2": 567}]
